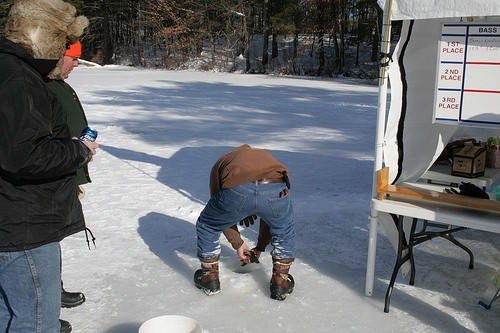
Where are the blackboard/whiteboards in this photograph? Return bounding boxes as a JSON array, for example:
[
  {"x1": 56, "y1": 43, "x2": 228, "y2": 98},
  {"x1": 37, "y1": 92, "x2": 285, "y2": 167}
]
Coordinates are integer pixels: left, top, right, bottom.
[{"x1": 432, "y1": 21, "x2": 500, "y2": 126}]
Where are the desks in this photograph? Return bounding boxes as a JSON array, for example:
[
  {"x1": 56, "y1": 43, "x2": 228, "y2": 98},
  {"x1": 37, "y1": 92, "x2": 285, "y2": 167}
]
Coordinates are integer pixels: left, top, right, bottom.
[{"x1": 370, "y1": 167, "x2": 500, "y2": 313}]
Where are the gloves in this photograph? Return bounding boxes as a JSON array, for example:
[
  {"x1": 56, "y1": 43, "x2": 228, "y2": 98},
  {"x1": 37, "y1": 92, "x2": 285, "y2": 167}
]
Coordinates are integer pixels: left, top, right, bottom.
[
  {"x1": 240, "y1": 214, "x2": 259, "y2": 227},
  {"x1": 239, "y1": 250, "x2": 260, "y2": 266},
  {"x1": 280, "y1": 189, "x2": 288, "y2": 198}
]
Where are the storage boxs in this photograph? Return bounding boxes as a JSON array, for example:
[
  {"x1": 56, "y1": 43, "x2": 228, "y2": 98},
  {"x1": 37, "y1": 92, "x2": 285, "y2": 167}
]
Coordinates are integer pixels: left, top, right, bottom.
[{"x1": 452, "y1": 147, "x2": 486, "y2": 178}]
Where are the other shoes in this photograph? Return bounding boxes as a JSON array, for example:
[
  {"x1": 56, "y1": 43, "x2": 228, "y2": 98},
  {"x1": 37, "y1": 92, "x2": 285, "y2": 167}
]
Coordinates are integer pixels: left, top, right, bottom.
[
  {"x1": 61, "y1": 288, "x2": 85, "y2": 308},
  {"x1": 59, "y1": 319, "x2": 72, "y2": 333}
]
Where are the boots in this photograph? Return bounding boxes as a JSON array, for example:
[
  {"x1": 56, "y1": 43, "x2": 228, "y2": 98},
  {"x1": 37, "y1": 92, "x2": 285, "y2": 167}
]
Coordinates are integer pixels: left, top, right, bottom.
[
  {"x1": 270, "y1": 261, "x2": 294, "y2": 301},
  {"x1": 193, "y1": 262, "x2": 221, "y2": 295}
]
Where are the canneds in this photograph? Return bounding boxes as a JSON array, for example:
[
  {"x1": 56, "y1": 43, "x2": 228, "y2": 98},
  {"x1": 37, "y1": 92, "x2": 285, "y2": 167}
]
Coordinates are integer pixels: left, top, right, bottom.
[{"x1": 78, "y1": 126, "x2": 98, "y2": 142}]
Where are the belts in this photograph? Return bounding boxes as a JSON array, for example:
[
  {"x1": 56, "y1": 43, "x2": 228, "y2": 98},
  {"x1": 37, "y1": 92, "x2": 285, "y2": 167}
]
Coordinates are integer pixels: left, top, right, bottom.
[{"x1": 254, "y1": 178, "x2": 283, "y2": 184}]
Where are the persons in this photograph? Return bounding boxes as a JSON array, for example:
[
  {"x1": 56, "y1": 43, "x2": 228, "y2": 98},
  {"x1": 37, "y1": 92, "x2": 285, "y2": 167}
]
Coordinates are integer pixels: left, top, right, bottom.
[
  {"x1": 194, "y1": 143, "x2": 295, "y2": 301},
  {"x1": 0, "y1": 0, "x2": 99, "y2": 333},
  {"x1": 46, "y1": 39, "x2": 92, "y2": 333}
]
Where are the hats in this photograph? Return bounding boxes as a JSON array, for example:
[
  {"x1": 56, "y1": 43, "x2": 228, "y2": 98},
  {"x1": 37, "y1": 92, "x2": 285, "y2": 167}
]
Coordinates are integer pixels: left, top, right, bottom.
[
  {"x1": 64, "y1": 37, "x2": 83, "y2": 58},
  {"x1": 5, "y1": 0, "x2": 90, "y2": 80}
]
